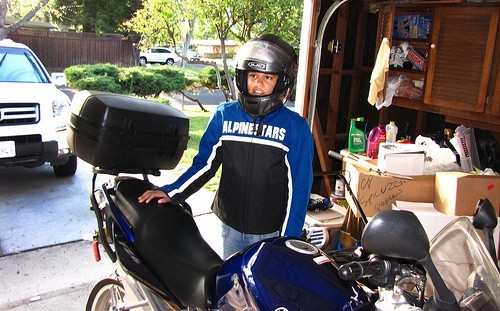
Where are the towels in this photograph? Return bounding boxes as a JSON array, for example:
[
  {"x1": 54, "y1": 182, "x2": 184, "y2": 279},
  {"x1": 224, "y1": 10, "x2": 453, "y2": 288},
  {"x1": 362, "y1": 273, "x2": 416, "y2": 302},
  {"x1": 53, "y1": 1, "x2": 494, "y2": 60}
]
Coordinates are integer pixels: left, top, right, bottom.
[{"x1": 368, "y1": 37, "x2": 391, "y2": 106}]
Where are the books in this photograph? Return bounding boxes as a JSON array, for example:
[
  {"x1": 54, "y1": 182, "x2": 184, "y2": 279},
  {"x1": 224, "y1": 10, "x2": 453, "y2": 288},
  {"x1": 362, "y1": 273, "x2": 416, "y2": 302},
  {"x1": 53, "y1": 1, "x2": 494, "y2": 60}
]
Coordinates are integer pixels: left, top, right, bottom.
[{"x1": 391, "y1": 15, "x2": 431, "y2": 71}]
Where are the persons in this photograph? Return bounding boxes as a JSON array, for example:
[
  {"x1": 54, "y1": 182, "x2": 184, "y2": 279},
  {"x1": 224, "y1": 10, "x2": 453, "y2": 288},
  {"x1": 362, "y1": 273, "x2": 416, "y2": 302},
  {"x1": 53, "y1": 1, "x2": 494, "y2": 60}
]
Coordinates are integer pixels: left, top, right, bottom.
[{"x1": 138, "y1": 34, "x2": 315, "y2": 262}]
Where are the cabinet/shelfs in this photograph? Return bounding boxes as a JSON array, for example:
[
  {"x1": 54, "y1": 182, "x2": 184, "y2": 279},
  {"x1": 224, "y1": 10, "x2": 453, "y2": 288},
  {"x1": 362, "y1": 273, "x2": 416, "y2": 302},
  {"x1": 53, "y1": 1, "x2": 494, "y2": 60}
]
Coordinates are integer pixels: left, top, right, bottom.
[{"x1": 374, "y1": 4, "x2": 500, "y2": 125}]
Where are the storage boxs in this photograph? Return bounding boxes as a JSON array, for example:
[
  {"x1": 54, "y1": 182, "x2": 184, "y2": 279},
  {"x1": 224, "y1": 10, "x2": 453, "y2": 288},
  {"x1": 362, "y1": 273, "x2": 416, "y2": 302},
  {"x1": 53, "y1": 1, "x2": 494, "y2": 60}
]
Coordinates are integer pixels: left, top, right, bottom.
[
  {"x1": 303, "y1": 203, "x2": 347, "y2": 240},
  {"x1": 407, "y1": 47, "x2": 426, "y2": 72},
  {"x1": 341, "y1": 141, "x2": 434, "y2": 216},
  {"x1": 65, "y1": 89, "x2": 190, "y2": 173},
  {"x1": 393, "y1": 14, "x2": 432, "y2": 40},
  {"x1": 433, "y1": 171, "x2": 500, "y2": 218}
]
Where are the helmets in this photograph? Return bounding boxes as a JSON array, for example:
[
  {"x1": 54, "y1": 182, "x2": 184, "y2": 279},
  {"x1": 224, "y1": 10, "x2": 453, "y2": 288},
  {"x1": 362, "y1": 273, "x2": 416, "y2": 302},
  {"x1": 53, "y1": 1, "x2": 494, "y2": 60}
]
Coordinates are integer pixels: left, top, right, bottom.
[{"x1": 233, "y1": 34, "x2": 297, "y2": 116}]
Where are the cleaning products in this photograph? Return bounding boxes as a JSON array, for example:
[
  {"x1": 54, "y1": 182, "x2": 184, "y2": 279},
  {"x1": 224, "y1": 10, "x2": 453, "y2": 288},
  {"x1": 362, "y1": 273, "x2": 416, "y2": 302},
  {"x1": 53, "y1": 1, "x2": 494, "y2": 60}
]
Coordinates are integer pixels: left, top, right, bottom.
[
  {"x1": 367, "y1": 124, "x2": 387, "y2": 159},
  {"x1": 348, "y1": 117, "x2": 367, "y2": 152},
  {"x1": 386, "y1": 121, "x2": 399, "y2": 142}
]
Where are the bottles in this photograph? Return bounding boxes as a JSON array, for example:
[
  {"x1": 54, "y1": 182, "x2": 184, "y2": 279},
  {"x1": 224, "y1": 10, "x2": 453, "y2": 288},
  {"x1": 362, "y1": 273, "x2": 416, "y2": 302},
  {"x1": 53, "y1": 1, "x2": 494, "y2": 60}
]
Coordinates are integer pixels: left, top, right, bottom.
[
  {"x1": 367, "y1": 124, "x2": 386, "y2": 159},
  {"x1": 386, "y1": 121, "x2": 398, "y2": 143}
]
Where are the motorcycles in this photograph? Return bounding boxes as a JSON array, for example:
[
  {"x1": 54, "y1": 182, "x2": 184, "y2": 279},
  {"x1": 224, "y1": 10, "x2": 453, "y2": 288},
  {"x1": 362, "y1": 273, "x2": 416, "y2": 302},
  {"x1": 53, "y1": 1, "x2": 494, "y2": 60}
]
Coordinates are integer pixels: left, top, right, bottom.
[{"x1": 66, "y1": 89, "x2": 500, "y2": 311}]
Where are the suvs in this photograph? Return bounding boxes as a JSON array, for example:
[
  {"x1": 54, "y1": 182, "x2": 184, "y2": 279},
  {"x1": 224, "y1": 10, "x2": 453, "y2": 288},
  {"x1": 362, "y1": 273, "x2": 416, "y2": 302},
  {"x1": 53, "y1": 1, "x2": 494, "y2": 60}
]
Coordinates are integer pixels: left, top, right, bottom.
[{"x1": 0, "y1": 37, "x2": 77, "y2": 178}]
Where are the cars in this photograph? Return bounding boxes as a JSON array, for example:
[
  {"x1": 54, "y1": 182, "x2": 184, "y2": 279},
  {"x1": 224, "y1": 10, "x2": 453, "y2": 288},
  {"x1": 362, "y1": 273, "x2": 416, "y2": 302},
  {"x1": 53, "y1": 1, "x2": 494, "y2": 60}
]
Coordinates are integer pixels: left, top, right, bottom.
[
  {"x1": 139, "y1": 46, "x2": 182, "y2": 66},
  {"x1": 187, "y1": 51, "x2": 202, "y2": 62}
]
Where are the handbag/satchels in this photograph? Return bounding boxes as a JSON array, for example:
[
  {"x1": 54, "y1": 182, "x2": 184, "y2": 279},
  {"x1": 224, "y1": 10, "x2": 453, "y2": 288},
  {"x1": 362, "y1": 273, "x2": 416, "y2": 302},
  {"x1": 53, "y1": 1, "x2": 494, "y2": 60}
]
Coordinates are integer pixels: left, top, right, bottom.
[{"x1": 449, "y1": 126, "x2": 481, "y2": 172}]
